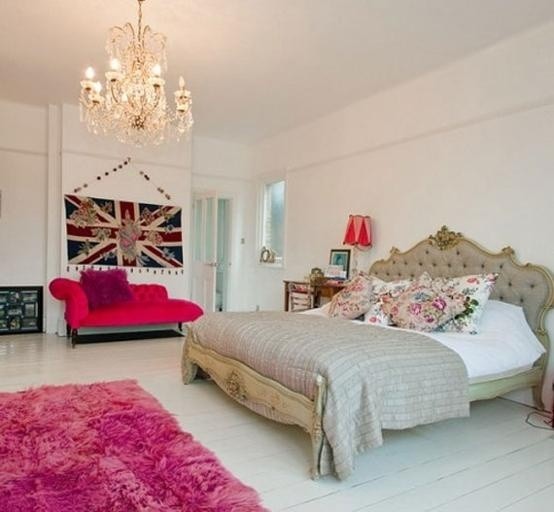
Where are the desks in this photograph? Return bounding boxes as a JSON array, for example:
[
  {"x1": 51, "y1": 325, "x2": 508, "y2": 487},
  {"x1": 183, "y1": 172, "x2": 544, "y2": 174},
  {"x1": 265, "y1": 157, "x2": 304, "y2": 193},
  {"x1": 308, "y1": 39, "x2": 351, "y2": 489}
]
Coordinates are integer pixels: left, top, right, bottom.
[{"x1": 282, "y1": 278, "x2": 346, "y2": 312}]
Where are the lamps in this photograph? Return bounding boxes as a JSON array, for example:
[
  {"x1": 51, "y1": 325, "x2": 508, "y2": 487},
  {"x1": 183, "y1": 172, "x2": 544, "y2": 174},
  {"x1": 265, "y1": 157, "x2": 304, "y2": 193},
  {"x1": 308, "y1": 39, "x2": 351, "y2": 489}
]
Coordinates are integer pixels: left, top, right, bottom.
[
  {"x1": 79, "y1": 0, "x2": 194, "y2": 148},
  {"x1": 342, "y1": 214, "x2": 373, "y2": 252}
]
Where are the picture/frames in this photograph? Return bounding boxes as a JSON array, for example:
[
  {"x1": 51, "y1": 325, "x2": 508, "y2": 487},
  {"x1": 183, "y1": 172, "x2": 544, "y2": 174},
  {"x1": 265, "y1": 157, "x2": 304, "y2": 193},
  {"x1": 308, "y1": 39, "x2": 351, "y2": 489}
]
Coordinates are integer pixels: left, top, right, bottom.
[{"x1": 329, "y1": 248, "x2": 351, "y2": 279}]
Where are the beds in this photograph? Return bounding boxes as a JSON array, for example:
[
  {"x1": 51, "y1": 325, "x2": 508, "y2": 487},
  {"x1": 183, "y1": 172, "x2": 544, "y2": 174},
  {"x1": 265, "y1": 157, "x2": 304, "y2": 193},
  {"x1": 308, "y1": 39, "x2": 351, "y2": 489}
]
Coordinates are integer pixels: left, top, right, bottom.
[{"x1": 182, "y1": 225, "x2": 554, "y2": 481}]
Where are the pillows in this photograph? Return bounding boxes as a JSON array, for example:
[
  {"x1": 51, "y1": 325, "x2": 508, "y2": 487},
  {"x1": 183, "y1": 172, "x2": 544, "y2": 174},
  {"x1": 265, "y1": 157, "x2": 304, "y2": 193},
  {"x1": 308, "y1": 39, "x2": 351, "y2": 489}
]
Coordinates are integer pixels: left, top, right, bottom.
[
  {"x1": 328, "y1": 271, "x2": 499, "y2": 336},
  {"x1": 79, "y1": 268, "x2": 133, "y2": 312}
]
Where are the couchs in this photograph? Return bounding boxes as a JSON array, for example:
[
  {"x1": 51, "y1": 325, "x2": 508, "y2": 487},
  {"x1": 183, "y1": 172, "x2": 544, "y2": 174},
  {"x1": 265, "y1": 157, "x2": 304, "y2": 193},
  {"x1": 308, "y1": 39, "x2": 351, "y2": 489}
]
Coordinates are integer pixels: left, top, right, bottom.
[{"x1": 48, "y1": 277, "x2": 204, "y2": 349}]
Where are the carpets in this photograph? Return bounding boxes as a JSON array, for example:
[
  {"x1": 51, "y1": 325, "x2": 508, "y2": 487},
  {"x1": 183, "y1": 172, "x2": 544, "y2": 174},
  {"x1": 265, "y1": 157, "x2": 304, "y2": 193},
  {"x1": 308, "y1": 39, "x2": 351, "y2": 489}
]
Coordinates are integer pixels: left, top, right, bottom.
[
  {"x1": 72, "y1": 329, "x2": 185, "y2": 344},
  {"x1": 0, "y1": 378, "x2": 272, "y2": 512}
]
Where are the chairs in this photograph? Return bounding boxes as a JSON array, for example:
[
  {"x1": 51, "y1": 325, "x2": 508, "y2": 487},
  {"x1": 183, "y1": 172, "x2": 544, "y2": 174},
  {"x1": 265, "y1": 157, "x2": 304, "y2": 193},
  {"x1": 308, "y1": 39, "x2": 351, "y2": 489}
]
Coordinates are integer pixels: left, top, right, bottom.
[{"x1": 288, "y1": 278, "x2": 314, "y2": 312}]
